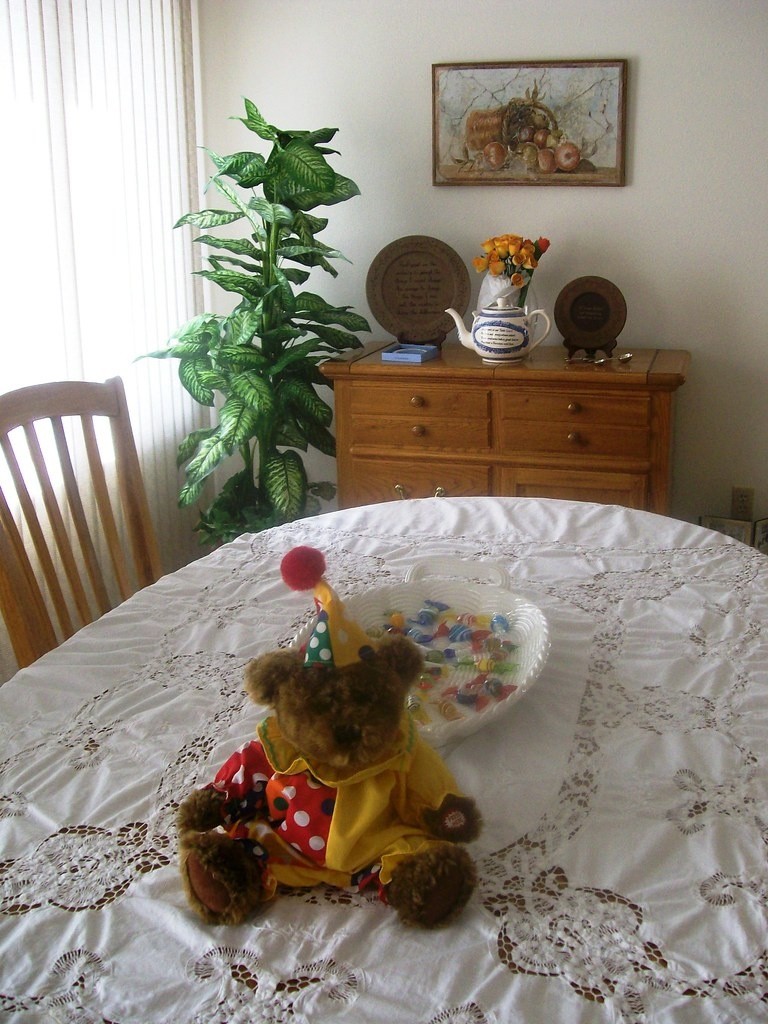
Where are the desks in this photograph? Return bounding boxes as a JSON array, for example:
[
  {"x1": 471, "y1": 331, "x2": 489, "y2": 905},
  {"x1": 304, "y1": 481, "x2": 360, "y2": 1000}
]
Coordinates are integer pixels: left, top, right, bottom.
[{"x1": 1, "y1": 497, "x2": 765, "y2": 1021}]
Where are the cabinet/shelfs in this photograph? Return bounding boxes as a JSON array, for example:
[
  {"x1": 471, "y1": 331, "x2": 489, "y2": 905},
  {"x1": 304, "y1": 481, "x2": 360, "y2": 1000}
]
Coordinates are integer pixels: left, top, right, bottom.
[{"x1": 319, "y1": 340, "x2": 692, "y2": 511}]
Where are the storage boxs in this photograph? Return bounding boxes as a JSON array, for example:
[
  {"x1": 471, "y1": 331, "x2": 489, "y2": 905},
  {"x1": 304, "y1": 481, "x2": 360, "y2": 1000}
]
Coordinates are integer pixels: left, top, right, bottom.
[{"x1": 382, "y1": 343, "x2": 438, "y2": 362}]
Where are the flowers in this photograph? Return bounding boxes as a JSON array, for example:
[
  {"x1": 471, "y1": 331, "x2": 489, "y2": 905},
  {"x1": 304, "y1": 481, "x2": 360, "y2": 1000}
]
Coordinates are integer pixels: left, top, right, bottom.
[{"x1": 471, "y1": 231, "x2": 550, "y2": 307}]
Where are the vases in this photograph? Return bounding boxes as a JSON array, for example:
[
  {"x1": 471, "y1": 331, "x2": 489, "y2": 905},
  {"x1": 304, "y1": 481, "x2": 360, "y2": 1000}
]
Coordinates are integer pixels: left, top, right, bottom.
[{"x1": 489, "y1": 280, "x2": 538, "y2": 340}]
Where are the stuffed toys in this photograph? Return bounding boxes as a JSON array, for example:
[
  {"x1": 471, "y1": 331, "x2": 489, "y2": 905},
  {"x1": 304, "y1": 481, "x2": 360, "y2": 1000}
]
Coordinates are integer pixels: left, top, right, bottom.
[{"x1": 176, "y1": 546, "x2": 480, "y2": 931}]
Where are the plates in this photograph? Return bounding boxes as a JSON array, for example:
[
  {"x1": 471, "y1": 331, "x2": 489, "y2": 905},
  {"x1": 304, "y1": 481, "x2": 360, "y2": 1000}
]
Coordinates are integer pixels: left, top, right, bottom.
[
  {"x1": 365, "y1": 234, "x2": 471, "y2": 343},
  {"x1": 553, "y1": 275, "x2": 627, "y2": 349},
  {"x1": 285, "y1": 577, "x2": 553, "y2": 747}
]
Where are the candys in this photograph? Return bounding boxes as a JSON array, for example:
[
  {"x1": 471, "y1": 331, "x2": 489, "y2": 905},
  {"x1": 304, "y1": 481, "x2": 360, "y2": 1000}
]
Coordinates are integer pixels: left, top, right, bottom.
[{"x1": 363, "y1": 598, "x2": 521, "y2": 725}]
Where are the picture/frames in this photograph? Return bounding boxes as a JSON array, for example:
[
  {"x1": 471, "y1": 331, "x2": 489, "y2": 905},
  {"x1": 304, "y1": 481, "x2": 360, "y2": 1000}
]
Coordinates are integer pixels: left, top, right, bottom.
[{"x1": 429, "y1": 60, "x2": 630, "y2": 190}]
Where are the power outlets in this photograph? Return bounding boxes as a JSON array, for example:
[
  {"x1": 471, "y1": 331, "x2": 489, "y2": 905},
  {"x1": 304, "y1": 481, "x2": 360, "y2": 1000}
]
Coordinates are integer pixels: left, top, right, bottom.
[{"x1": 730, "y1": 485, "x2": 757, "y2": 520}]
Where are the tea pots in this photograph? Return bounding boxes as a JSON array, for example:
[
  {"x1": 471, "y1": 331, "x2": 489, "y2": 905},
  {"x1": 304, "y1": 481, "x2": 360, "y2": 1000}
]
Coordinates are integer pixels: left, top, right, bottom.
[{"x1": 444, "y1": 297, "x2": 551, "y2": 364}]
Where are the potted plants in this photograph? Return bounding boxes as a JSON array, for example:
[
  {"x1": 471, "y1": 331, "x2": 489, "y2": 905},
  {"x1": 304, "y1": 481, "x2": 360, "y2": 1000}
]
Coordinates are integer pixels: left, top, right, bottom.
[{"x1": 129, "y1": 94, "x2": 372, "y2": 551}]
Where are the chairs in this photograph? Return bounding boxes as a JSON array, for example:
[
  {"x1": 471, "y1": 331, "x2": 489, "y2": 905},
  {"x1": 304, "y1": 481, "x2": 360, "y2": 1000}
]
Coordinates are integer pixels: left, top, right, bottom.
[{"x1": 0, "y1": 376, "x2": 162, "y2": 671}]
